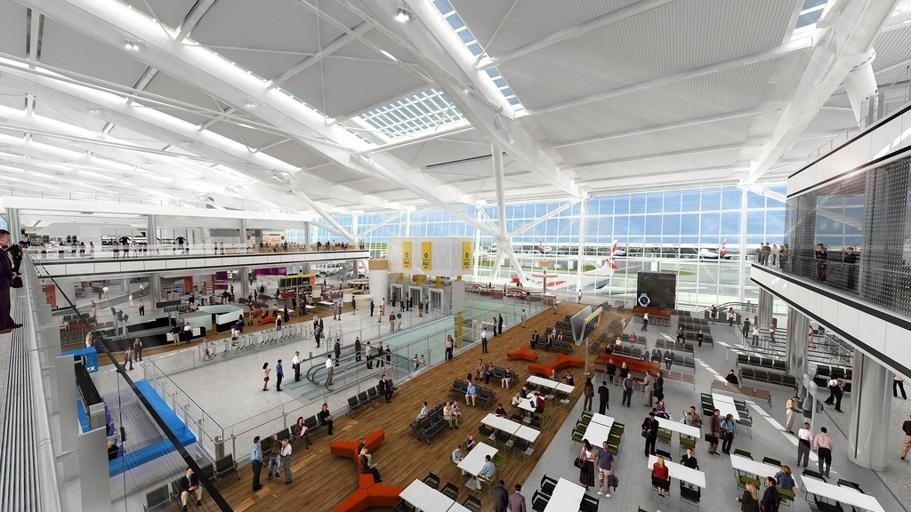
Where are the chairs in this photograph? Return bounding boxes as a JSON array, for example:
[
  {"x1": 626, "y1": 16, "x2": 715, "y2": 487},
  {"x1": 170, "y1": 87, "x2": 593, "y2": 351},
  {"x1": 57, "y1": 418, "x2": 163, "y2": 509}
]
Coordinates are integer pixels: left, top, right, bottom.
[
  {"x1": 528, "y1": 473, "x2": 601, "y2": 512},
  {"x1": 346, "y1": 379, "x2": 399, "y2": 421},
  {"x1": 510, "y1": 390, "x2": 547, "y2": 430},
  {"x1": 530, "y1": 297, "x2": 611, "y2": 353},
  {"x1": 463, "y1": 285, "x2": 557, "y2": 307},
  {"x1": 816, "y1": 326, "x2": 855, "y2": 363},
  {"x1": 407, "y1": 399, "x2": 456, "y2": 447},
  {"x1": 811, "y1": 363, "x2": 852, "y2": 394},
  {"x1": 704, "y1": 309, "x2": 778, "y2": 328},
  {"x1": 698, "y1": 391, "x2": 755, "y2": 439},
  {"x1": 328, "y1": 428, "x2": 402, "y2": 511},
  {"x1": 103, "y1": 400, "x2": 125, "y2": 462},
  {"x1": 257, "y1": 409, "x2": 333, "y2": 463},
  {"x1": 449, "y1": 438, "x2": 510, "y2": 496},
  {"x1": 447, "y1": 377, "x2": 498, "y2": 412},
  {"x1": 475, "y1": 361, "x2": 519, "y2": 391},
  {"x1": 645, "y1": 448, "x2": 706, "y2": 508},
  {"x1": 393, "y1": 471, "x2": 481, "y2": 511},
  {"x1": 728, "y1": 447, "x2": 797, "y2": 508},
  {"x1": 733, "y1": 351, "x2": 799, "y2": 392},
  {"x1": 590, "y1": 306, "x2": 714, "y2": 394},
  {"x1": 477, "y1": 409, "x2": 542, "y2": 465},
  {"x1": 253, "y1": 241, "x2": 355, "y2": 253},
  {"x1": 140, "y1": 451, "x2": 240, "y2": 512},
  {"x1": 526, "y1": 374, "x2": 576, "y2": 407},
  {"x1": 527, "y1": 351, "x2": 585, "y2": 378},
  {"x1": 104, "y1": 286, "x2": 367, "y2": 352},
  {"x1": 506, "y1": 345, "x2": 539, "y2": 361},
  {"x1": 654, "y1": 410, "x2": 703, "y2": 452},
  {"x1": 569, "y1": 409, "x2": 626, "y2": 461},
  {"x1": 710, "y1": 378, "x2": 773, "y2": 408}
]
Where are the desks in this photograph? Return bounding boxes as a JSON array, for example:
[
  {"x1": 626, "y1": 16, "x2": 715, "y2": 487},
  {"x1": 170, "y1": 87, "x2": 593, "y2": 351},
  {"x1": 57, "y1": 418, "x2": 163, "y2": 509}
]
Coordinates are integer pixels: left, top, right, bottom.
[{"x1": 799, "y1": 474, "x2": 884, "y2": 512}]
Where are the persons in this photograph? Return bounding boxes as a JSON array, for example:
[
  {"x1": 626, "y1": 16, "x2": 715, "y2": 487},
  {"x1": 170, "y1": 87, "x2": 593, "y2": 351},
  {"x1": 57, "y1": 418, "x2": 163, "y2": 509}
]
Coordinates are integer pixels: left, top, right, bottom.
[
  {"x1": 578, "y1": 289, "x2": 582, "y2": 304},
  {"x1": 202, "y1": 339, "x2": 216, "y2": 361},
  {"x1": 901, "y1": 415, "x2": 911, "y2": 460},
  {"x1": 521, "y1": 309, "x2": 526, "y2": 328},
  {"x1": 532, "y1": 326, "x2": 557, "y2": 351},
  {"x1": 27, "y1": 235, "x2": 94, "y2": 256},
  {"x1": 379, "y1": 374, "x2": 393, "y2": 404},
  {"x1": 416, "y1": 361, "x2": 669, "y2": 512},
  {"x1": 178, "y1": 466, "x2": 202, "y2": 511},
  {"x1": 260, "y1": 240, "x2": 287, "y2": 252},
  {"x1": 358, "y1": 438, "x2": 382, "y2": 484},
  {"x1": 482, "y1": 314, "x2": 503, "y2": 354},
  {"x1": 128, "y1": 293, "x2": 134, "y2": 305},
  {"x1": 250, "y1": 403, "x2": 333, "y2": 492},
  {"x1": 678, "y1": 368, "x2": 844, "y2": 511},
  {"x1": 359, "y1": 240, "x2": 365, "y2": 250},
  {"x1": 170, "y1": 322, "x2": 193, "y2": 346},
  {"x1": 85, "y1": 301, "x2": 145, "y2": 371},
  {"x1": 102, "y1": 236, "x2": 135, "y2": 257},
  {"x1": 753, "y1": 237, "x2": 856, "y2": 289},
  {"x1": 609, "y1": 310, "x2": 703, "y2": 371},
  {"x1": 80, "y1": 281, "x2": 109, "y2": 299},
  {"x1": 807, "y1": 328, "x2": 815, "y2": 346},
  {"x1": 8, "y1": 240, "x2": 25, "y2": 288},
  {"x1": 244, "y1": 279, "x2": 429, "y2": 392},
  {"x1": 445, "y1": 335, "x2": 454, "y2": 362},
  {"x1": 223, "y1": 314, "x2": 245, "y2": 352},
  {"x1": 708, "y1": 305, "x2": 774, "y2": 346},
  {"x1": 0, "y1": 229, "x2": 24, "y2": 335},
  {"x1": 317, "y1": 239, "x2": 349, "y2": 251},
  {"x1": 173, "y1": 237, "x2": 188, "y2": 247},
  {"x1": 893, "y1": 373, "x2": 907, "y2": 400},
  {"x1": 140, "y1": 282, "x2": 144, "y2": 295},
  {"x1": 184, "y1": 283, "x2": 233, "y2": 313}
]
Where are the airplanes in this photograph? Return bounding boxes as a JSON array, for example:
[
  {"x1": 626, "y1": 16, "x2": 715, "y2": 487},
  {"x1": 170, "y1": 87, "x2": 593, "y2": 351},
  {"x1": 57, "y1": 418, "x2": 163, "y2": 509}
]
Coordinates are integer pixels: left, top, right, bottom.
[
  {"x1": 449, "y1": 239, "x2": 706, "y2": 295},
  {"x1": 603, "y1": 247, "x2": 634, "y2": 256},
  {"x1": 677, "y1": 239, "x2": 740, "y2": 260},
  {"x1": 516, "y1": 239, "x2": 567, "y2": 254}
]
